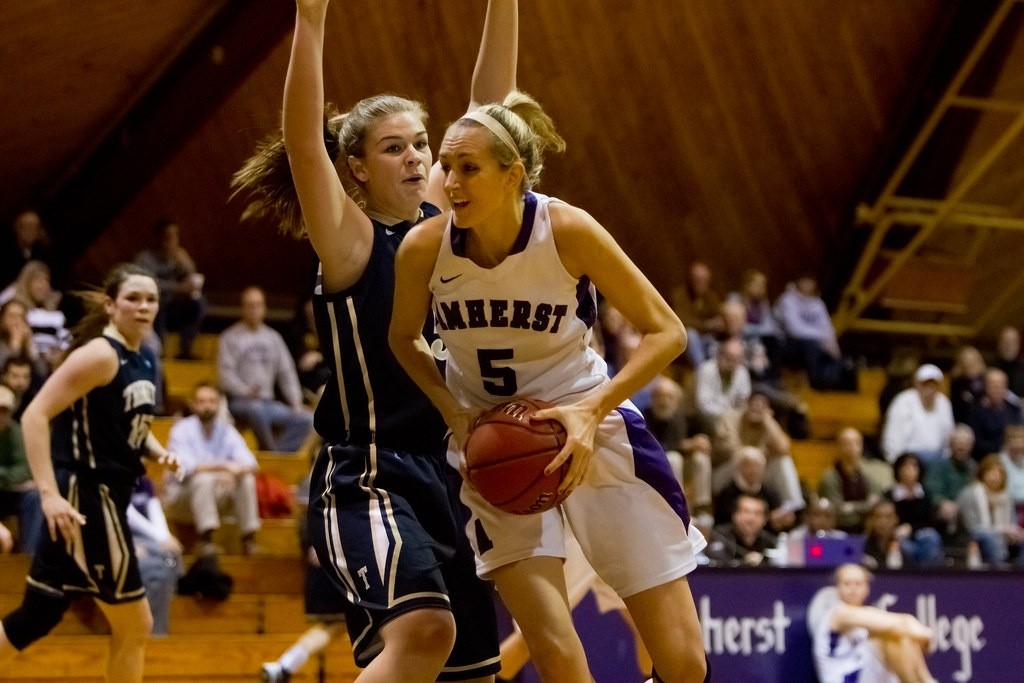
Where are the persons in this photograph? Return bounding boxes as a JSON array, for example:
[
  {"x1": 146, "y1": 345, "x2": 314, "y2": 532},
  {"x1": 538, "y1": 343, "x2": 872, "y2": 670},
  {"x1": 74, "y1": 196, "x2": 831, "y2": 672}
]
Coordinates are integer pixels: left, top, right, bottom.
[
  {"x1": 0, "y1": 263, "x2": 183, "y2": 682},
  {"x1": 387, "y1": 93, "x2": 711, "y2": 683},
  {"x1": 812, "y1": 565, "x2": 939, "y2": 683},
  {"x1": 590, "y1": 261, "x2": 1024, "y2": 569},
  {"x1": 229, "y1": 1, "x2": 518, "y2": 683},
  {"x1": 0, "y1": 213, "x2": 315, "y2": 554}
]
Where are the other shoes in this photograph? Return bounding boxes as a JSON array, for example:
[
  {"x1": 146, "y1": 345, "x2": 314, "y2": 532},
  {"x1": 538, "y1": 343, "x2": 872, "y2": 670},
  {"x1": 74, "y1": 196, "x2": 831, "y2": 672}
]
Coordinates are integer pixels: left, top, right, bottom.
[
  {"x1": 239, "y1": 539, "x2": 257, "y2": 554},
  {"x1": 200, "y1": 541, "x2": 226, "y2": 555}
]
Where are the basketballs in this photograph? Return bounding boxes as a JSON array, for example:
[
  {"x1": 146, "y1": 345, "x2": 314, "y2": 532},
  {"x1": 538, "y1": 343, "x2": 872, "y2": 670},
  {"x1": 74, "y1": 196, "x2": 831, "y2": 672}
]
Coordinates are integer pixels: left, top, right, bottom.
[{"x1": 464, "y1": 396, "x2": 583, "y2": 516}]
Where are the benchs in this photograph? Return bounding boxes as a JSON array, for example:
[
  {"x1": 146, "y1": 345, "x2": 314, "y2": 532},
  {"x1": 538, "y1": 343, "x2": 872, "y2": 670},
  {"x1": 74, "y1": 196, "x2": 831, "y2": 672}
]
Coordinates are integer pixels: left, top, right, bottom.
[{"x1": 0, "y1": 303, "x2": 1024, "y2": 683}]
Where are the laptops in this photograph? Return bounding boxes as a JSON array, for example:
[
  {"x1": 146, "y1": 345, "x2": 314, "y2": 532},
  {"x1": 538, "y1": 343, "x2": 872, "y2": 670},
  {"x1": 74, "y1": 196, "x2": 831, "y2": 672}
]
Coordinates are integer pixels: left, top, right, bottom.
[{"x1": 804, "y1": 537, "x2": 866, "y2": 568}]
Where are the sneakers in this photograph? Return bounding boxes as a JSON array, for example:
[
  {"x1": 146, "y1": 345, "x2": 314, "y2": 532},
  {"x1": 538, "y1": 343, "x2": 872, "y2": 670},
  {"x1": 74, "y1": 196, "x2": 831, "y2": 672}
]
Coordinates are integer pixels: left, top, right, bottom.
[{"x1": 261, "y1": 661, "x2": 293, "y2": 682}]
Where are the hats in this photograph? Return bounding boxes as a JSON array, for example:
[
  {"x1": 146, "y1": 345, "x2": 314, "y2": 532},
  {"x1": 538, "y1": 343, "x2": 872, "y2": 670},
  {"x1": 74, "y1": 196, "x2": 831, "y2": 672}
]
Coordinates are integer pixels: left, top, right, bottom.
[{"x1": 915, "y1": 362, "x2": 942, "y2": 384}]
